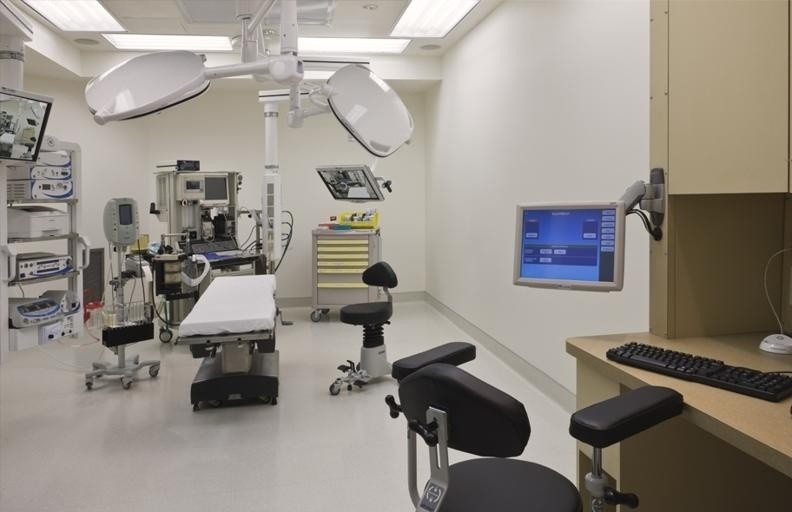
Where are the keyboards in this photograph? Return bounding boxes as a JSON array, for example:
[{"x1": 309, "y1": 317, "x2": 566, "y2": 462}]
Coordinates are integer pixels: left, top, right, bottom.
[{"x1": 605, "y1": 339, "x2": 791, "y2": 405}]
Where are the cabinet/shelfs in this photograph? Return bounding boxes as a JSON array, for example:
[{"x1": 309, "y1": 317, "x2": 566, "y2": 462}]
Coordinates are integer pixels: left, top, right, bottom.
[
  {"x1": 311, "y1": 228, "x2": 382, "y2": 324},
  {"x1": 7, "y1": 198, "x2": 81, "y2": 329},
  {"x1": 666, "y1": 0, "x2": 792, "y2": 196}
]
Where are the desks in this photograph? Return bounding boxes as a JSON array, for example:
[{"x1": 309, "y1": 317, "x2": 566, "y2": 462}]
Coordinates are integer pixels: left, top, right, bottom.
[
  {"x1": 182, "y1": 254, "x2": 267, "y2": 320},
  {"x1": 562, "y1": 333, "x2": 792, "y2": 512}
]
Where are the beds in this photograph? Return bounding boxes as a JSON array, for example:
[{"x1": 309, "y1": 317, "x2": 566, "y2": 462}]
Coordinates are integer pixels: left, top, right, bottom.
[{"x1": 175, "y1": 273, "x2": 282, "y2": 411}]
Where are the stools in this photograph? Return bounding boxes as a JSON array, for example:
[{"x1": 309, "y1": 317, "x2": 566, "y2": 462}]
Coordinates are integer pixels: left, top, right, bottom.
[{"x1": 84, "y1": 317, "x2": 161, "y2": 389}]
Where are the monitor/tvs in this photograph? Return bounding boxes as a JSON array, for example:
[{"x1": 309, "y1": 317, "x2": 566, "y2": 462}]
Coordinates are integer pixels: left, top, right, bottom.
[
  {"x1": 72, "y1": 248, "x2": 105, "y2": 309},
  {"x1": 199, "y1": 174, "x2": 229, "y2": 206},
  {"x1": 314, "y1": 165, "x2": 385, "y2": 202},
  {"x1": 512, "y1": 201, "x2": 627, "y2": 293},
  {"x1": 0, "y1": 85, "x2": 55, "y2": 162}
]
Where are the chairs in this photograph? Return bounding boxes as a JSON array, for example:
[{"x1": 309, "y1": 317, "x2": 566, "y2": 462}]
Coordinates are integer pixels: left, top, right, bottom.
[
  {"x1": 327, "y1": 260, "x2": 393, "y2": 395},
  {"x1": 384, "y1": 340, "x2": 686, "y2": 512}
]
